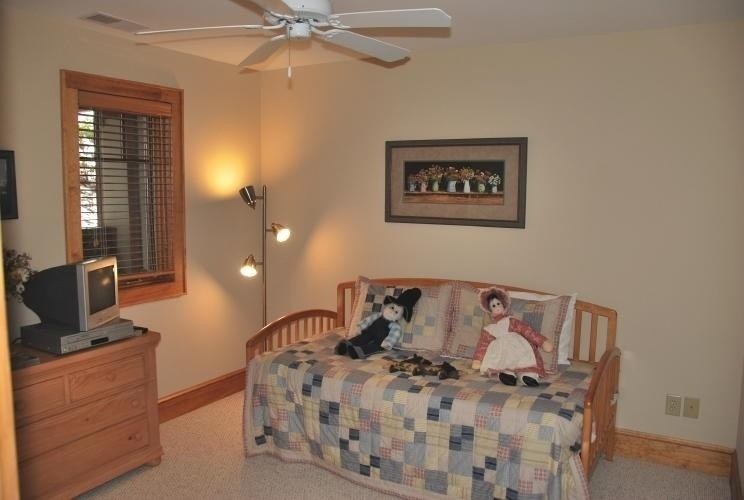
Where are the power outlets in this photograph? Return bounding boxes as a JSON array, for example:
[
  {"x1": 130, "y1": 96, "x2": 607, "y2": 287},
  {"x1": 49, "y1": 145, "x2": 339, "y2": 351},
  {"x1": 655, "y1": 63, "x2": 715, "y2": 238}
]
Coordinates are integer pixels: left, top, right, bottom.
[{"x1": 665, "y1": 394, "x2": 682, "y2": 418}]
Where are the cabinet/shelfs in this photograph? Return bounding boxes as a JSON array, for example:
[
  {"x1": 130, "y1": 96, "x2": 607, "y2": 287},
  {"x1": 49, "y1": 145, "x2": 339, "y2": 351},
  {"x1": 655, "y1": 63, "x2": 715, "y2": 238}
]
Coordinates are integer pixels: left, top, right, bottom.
[{"x1": 7, "y1": 325, "x2": 166, "y2": 500}]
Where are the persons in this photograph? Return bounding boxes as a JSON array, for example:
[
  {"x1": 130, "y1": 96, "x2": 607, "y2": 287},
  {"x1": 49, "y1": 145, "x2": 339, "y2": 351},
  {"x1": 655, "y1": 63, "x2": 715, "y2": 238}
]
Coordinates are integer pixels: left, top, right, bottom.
[
  {"x1": 335, "y1": 288, "x2": 421, "y2": 359},
  {"x1": 471, "y1": 288, "x2": 553, "y2": 387}
]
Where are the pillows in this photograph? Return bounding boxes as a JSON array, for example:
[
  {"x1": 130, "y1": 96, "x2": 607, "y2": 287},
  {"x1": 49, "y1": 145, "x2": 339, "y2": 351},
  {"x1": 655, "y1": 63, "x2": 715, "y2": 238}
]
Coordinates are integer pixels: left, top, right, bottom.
[
  {"x1": 339, "y1": 274, "x2": 458, "y2": 359},
  {"x1": 470, "y1": 284, "x2": 581, "y2": 369},
  {"x1": 443, "y1": 278, "x2": 570, "y2": 375}
]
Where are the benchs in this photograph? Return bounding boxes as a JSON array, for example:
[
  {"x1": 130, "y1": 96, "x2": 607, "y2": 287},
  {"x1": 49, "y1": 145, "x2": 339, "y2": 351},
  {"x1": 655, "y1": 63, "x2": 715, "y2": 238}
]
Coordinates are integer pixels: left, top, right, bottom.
[{"x1": 243, "y1": 276, "x2": 623, "y2": 499}]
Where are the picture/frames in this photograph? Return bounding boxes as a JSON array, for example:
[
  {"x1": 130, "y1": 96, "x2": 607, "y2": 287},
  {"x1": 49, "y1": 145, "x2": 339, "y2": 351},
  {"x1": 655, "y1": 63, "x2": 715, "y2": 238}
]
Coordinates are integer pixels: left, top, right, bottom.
[
  {"x1": 381, "y1": 134, "x2": 531, "y2": 230},
  {"x1": 0, "y1": 149, "x2": 20, "y2": 222}
]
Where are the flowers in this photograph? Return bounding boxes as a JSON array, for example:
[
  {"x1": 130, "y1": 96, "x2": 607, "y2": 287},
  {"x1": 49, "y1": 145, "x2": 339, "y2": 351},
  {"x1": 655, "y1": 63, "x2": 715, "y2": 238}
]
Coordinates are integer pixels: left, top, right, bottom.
[
  {"x1": 1, "y1": 241, "x2": 34, "y2": 306},
  {"x1": 407, "y1": 162, "x2": 501, "y2": 194}
]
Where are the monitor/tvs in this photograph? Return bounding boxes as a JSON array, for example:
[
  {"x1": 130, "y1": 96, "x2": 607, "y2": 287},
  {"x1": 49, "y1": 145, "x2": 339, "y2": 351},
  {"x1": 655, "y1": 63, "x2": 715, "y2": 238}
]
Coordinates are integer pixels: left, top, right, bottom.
[{"x1": 22, "y1": 256, "x2": 120, "y2": 332}]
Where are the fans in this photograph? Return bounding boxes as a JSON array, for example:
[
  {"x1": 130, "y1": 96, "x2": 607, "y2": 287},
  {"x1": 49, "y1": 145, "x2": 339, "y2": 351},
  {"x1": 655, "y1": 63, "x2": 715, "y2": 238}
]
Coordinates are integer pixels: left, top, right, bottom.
[{"x1": 130, "y1": 0, "x2": 457, "y2": 82}]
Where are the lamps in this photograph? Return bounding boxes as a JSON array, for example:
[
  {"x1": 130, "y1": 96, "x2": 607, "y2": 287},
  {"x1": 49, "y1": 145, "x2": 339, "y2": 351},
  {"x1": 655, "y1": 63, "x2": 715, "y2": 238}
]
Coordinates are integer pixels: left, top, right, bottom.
[{"x1": 233, "y1": 183, "x2": 292, "y2": 358}]
